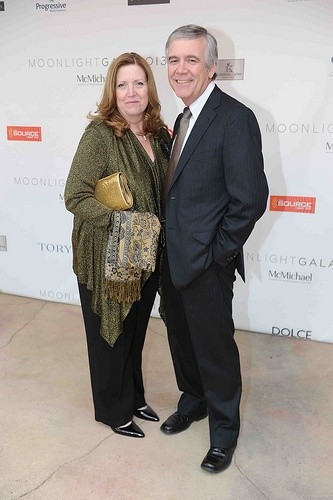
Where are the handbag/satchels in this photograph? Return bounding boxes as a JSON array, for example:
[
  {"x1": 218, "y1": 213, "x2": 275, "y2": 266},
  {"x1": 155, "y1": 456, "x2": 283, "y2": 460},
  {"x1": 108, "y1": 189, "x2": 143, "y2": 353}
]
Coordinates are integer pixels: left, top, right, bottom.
[{"x1": 94, "y1": 171, "x2": 134, "y2": 211}]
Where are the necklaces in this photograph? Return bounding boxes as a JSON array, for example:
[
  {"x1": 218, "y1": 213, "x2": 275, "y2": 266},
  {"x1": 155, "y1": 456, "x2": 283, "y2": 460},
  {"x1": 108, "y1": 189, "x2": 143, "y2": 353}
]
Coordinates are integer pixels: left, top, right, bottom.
[{"x1": 130, "y1": 128, "x2": 147, "y2": 141}]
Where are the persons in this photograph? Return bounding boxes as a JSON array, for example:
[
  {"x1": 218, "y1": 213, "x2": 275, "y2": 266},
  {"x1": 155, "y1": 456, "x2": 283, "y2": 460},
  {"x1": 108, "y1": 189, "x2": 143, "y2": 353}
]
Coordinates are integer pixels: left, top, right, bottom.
[
  {"x1": 64, "y1": 52, "x2": 173, "y2": 437},
  {"x1": 160, "y1": 25, "x2": 268, "y2": 473}
]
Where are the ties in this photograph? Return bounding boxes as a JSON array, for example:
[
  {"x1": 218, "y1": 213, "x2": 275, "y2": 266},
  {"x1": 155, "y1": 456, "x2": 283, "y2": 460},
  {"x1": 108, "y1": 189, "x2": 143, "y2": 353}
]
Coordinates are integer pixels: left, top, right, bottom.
[{"x1": 161, "y1": 107, "x2": 192, "y2": 208}]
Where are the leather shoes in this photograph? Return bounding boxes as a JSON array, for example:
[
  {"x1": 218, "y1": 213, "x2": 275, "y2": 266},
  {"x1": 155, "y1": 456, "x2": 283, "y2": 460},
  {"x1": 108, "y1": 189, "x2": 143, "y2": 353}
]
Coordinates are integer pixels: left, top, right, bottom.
[
  {"x1": 199, "y1": 446, "x2": 237, "y2": 473},
  {"x1": 133, "y1": 404, "x2": 160, "y2": 421},
  {"x1": 95, "y1": 417, "x2": 145, "y2": 438},
  {"x1": 160, "y1": 412, "x2": 208, "y2": 434}
]
